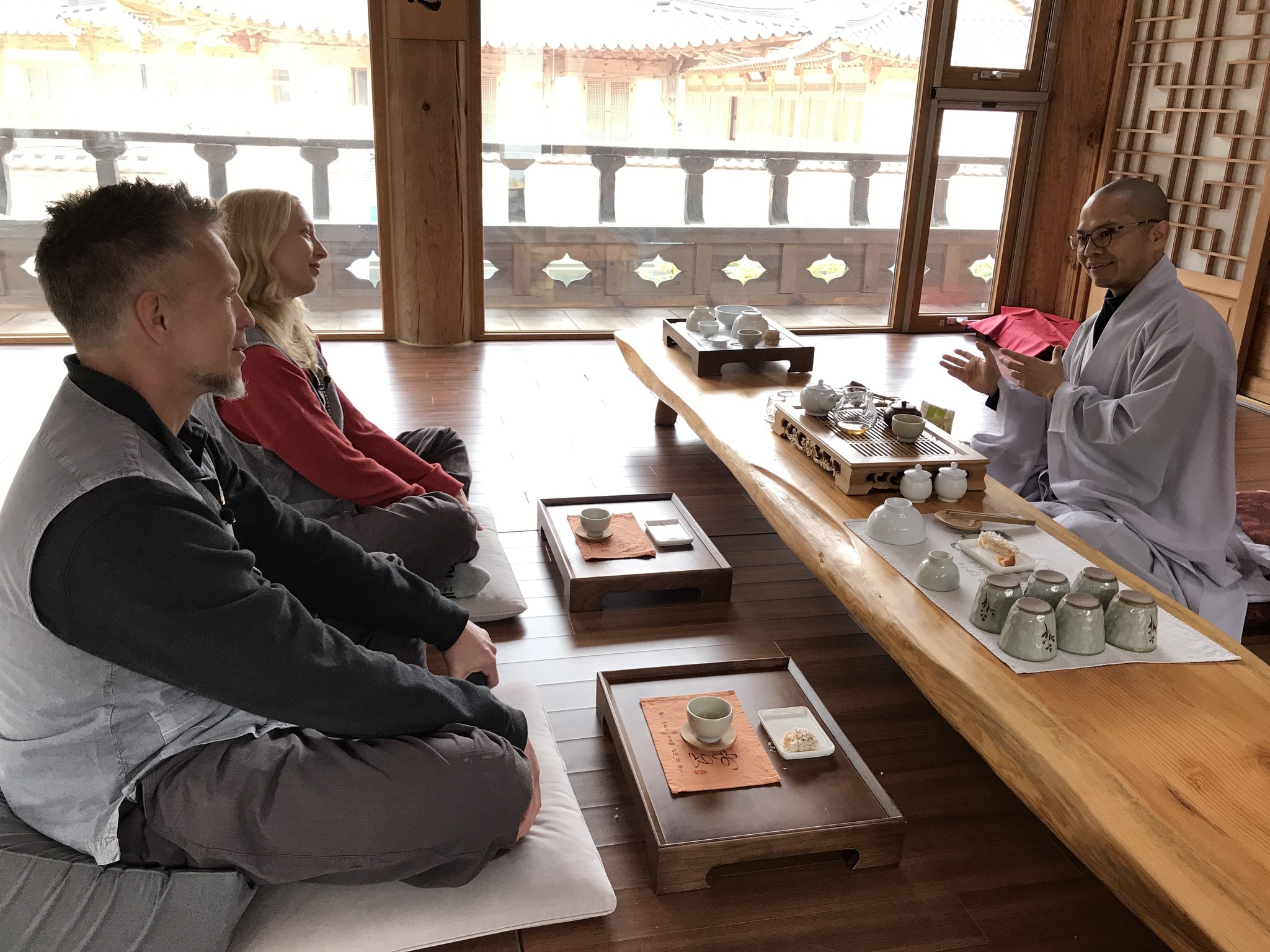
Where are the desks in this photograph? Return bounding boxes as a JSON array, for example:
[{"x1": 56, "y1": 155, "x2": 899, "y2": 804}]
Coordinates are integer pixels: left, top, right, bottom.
[{"x1": 608, "y1": 318, "x2": 1269, "y2": 952}]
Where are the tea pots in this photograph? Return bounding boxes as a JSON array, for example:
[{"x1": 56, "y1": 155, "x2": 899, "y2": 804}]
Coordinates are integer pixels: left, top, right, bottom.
[
  {"x1": 801, "y1": 378, "x2": 840, "y2": 416},
  {"x1": 884, "y1": 399, "x2": 922, "y2": 430}
]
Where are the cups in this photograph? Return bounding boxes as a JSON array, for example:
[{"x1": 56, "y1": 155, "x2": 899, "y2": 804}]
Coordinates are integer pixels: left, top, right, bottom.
[
  {"x1": 580, "y1": 508, "x2": 612, "y2": 537},
  {"x1": 764, "y1": 395, "x2": 786, "y2": 422},
  {"x1": 935, "y1": 462, "x2": 967, "y2": 503},
  {"x1": 916, "y1": 550, "x2": 960, "y2": 592},
  {"x1": 971, "y1": 567, "x2": 1159, "y2": 662},
  {"x1": 900, "y1": 464, "x2": 932, "y2": 504},
  {"x1": 762, "y1": 329, "x2": 780, "y2": 346},
  {"x1": 777, "y1": 390, "x2": 795, "y2": 403},
  {"x1": 687, "y1": 695, "x2": 733, "y2": 743},
  {"x1": 891, "y1": 414, "x2": 926, "y2": 443}
]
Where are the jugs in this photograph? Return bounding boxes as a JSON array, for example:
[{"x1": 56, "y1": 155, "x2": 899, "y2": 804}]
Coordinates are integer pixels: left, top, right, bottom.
[{"x1": 834, "y1": 385, "x2": 879, "y2": 435}]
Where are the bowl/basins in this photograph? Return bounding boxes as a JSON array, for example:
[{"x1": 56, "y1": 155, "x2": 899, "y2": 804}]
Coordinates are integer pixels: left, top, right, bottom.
[
  {"x1": 737, "y1": 329, "x2": 762, "y2": 349},
  {"x1": 685, "y1": 305, "x2": 715, "y2": 331},
  {"x1": 866, "y1": 498, "x2": 927, "y2": 546},
  {"x1": 730, "y1": 310, "x2": 769, "y2": 340},
  {"x1": 714, "y1": 304, "x2": 758, "y2": 328},
  {"x1": 698, "y1": 320, "x2": 720, "y2": 338}
]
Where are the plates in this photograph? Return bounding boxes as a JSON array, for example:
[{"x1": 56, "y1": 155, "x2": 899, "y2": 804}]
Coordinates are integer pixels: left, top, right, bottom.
[
  {"x1": 644, "y1": 518, "x2": 694, "y2": 547},
  {"x1": 576, "y1": 525, "x2": 614, "y2": 540},
  {"x1": 958, "y1": 537, "x2": 1039, "y2": 574},
  {"x1": 935, "y1": 509, "x2": 983, "y2": 531},
  {"x1": 680, "y1": 719, "x2": 737, "y2": 751},
  {"x1": 758, "y1": 705, "x2": 836, "y2": 759}
]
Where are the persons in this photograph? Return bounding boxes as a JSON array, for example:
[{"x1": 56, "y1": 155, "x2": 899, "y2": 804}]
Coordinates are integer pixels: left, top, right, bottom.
[
  {"x1": 0, "y1": 176, "x2": 542, "y2": 888},
  {"x1": 190, "y1": 188, "x2": 491, "y2": 599},
  {"x1": 940, "y1": 179, "x2": 1270, "y2": 644}
]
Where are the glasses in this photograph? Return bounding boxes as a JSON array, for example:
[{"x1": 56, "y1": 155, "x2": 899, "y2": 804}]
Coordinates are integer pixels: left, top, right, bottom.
[{"x1": 1066, "y1": 220, "x2": 1160, "y2": 254}]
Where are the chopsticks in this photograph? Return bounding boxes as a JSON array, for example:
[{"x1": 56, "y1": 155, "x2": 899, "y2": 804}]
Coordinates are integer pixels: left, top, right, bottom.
[{"x1": 945, "y1": 508, "x2": 1036, "y2": 526}]
